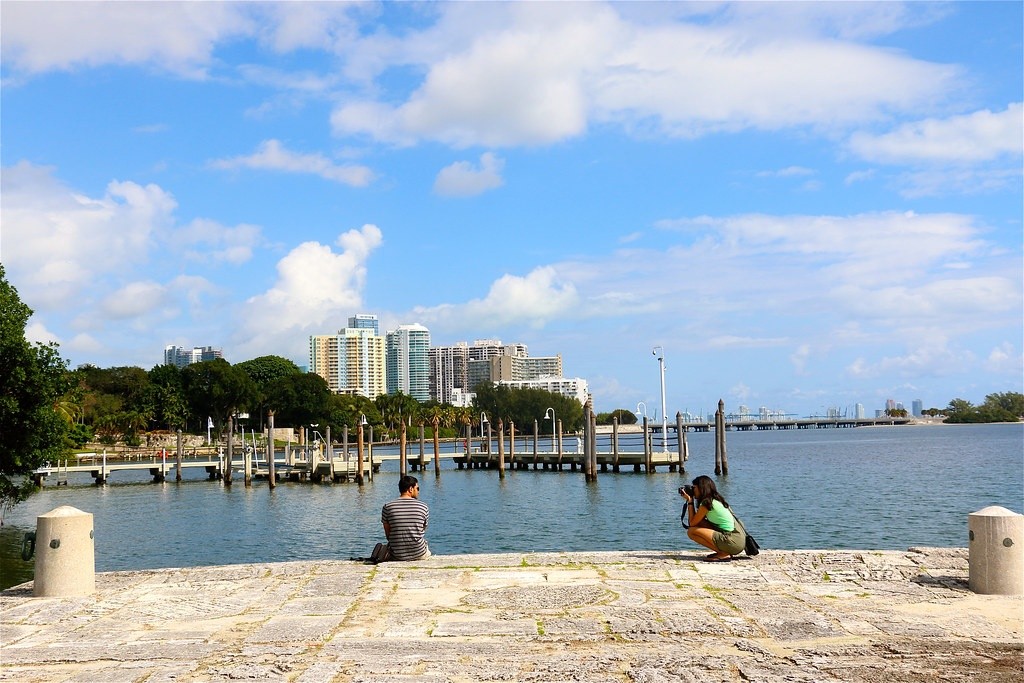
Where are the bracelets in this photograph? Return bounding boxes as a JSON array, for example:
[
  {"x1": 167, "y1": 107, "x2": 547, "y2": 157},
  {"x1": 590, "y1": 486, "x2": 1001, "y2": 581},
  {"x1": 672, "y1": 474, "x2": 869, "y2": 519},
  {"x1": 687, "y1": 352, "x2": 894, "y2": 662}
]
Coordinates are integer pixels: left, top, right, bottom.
[{"x1": 688, "y1": 502, "x2": 693, "y2": 505}]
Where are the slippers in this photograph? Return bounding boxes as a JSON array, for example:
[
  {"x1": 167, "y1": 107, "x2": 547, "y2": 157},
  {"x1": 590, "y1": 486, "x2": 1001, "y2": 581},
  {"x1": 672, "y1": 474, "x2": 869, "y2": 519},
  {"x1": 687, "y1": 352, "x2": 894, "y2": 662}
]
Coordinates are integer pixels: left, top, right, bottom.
[
  {"x1": 707, "y1": 553, "x2": 733, "y2": 559},
  {"x1": 703, "y1": 556, "x2": 731, "y2": 562}
]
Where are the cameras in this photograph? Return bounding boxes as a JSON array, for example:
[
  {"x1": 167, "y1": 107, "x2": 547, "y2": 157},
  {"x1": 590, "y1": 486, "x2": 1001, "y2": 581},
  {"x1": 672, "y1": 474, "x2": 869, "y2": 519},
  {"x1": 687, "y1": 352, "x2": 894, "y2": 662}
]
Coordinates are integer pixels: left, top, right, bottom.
[{"x1": 678, "y1": 484, "x2": 695, "y2": 498}]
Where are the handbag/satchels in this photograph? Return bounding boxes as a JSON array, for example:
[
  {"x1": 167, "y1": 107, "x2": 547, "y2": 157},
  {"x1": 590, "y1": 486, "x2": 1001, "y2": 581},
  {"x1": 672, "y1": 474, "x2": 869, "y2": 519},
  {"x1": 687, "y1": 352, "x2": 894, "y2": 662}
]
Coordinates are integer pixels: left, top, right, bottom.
[{"x1": 744, "y1": 535, "x2": 760, "y2": 556}]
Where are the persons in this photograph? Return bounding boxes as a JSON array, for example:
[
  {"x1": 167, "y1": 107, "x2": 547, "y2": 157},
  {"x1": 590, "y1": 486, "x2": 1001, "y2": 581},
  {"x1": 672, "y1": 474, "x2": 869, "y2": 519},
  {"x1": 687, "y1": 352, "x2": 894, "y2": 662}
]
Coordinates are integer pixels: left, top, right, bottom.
[
  {"x1": 380, "y1": 475, "x2": 432, "y2": 560},
  {"x1": 680, "y1": 475, "x2": 747, "y2": 562}
]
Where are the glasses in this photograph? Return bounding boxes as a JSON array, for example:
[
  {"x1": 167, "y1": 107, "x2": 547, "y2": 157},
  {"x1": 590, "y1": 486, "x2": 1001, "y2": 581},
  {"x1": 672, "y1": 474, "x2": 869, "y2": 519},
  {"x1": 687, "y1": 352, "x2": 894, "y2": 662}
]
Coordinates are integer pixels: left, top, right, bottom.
[{"x1": 416, "y1": 487, "x2": 419, "y2": 491}]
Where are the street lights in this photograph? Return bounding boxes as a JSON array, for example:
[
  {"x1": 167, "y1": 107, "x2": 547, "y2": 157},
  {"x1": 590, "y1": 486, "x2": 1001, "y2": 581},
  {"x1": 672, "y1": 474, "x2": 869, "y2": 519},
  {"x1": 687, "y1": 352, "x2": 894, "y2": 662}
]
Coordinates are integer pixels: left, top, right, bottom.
[
  {"x1": 652, "y1": 345, "x2": 668, "y2": 451},
  {"x1": 544, "y1": 407, "x2": 556, "y2": 452},
  {"x1": 208, "y1": 417, "x2": 214, "y2": 445},
  {"x1": 480, "y1": 412, "x2": 488, "y2": 451}
]
